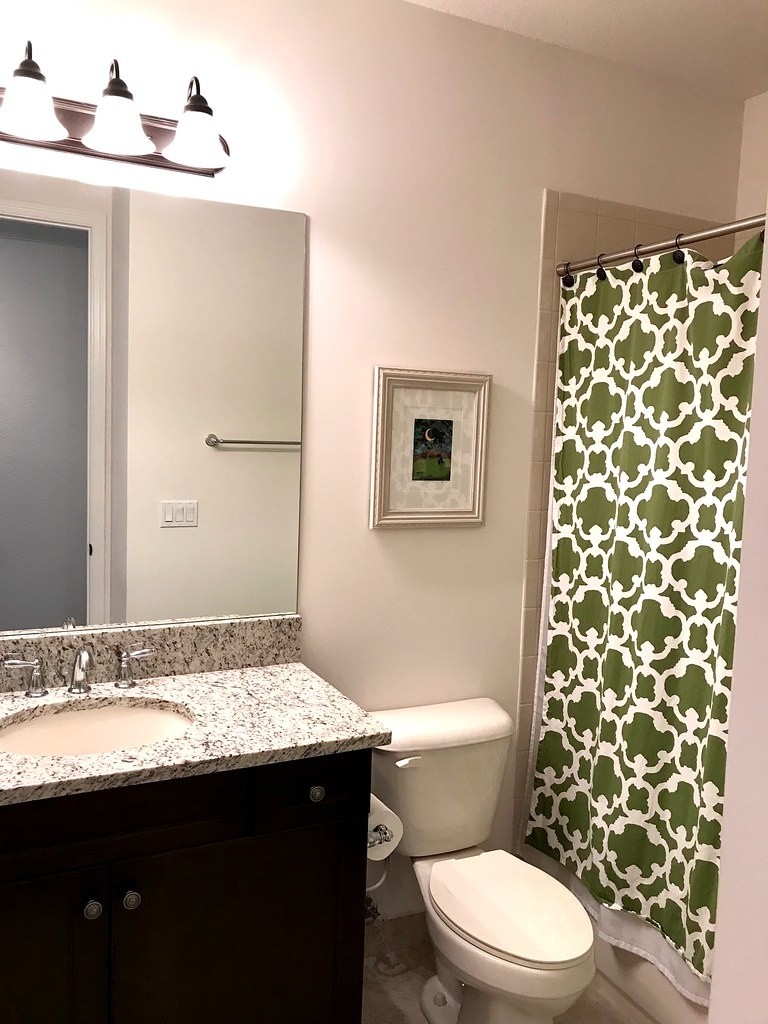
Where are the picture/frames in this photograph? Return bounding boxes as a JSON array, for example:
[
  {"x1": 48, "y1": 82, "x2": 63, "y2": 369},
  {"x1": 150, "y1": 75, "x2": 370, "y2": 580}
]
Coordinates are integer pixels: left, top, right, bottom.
[{"x1": 366, "y1": 367, "x2": 493, "y2": 529}]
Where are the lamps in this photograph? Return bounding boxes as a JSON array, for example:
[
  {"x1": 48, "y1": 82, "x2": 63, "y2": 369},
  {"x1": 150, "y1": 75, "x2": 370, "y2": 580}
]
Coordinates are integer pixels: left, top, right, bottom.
[
  {"x1": 161, "y1": 77, "x2": 235, "y2": 167},
  {"x1": 80, "y1": 59, "x2": 154, "y2": 156},
  {"x1": 0, "y1": 42, "x2": 66, "y2": 142}
]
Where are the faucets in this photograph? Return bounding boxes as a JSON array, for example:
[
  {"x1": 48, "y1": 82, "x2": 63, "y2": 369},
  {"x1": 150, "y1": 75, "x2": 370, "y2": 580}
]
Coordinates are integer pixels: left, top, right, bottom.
[
  {"x1": 68, "y1": 650, "x2": 95, "y2": 694},
  {"x1": 62, "y1": 616, "x2": 77, "y2": 631}
]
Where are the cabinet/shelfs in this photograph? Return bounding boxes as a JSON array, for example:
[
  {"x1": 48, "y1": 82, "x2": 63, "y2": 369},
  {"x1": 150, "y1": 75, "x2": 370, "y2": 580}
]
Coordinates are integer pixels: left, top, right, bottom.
[{"x1": 0, "y1": 751, "x2": 370, "y2": 1024}]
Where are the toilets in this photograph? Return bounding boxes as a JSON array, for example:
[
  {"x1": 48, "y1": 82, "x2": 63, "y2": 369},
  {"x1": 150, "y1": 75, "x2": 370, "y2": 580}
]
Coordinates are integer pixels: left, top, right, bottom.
[{"x1": 358, "y1": 697, "x2": 598, "y2": 1024}]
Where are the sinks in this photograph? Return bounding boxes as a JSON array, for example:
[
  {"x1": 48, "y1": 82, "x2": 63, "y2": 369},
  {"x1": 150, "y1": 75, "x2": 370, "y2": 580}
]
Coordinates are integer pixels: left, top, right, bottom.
[{"x1": 0, "y1": 696, "x2": 197, "y2": 755}]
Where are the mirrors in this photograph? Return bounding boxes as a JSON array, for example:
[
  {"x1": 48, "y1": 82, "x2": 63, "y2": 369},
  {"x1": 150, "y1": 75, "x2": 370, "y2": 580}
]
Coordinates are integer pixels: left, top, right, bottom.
[{"x1": 0, "y1": 165, "x2": 310, "y2": 638}]
